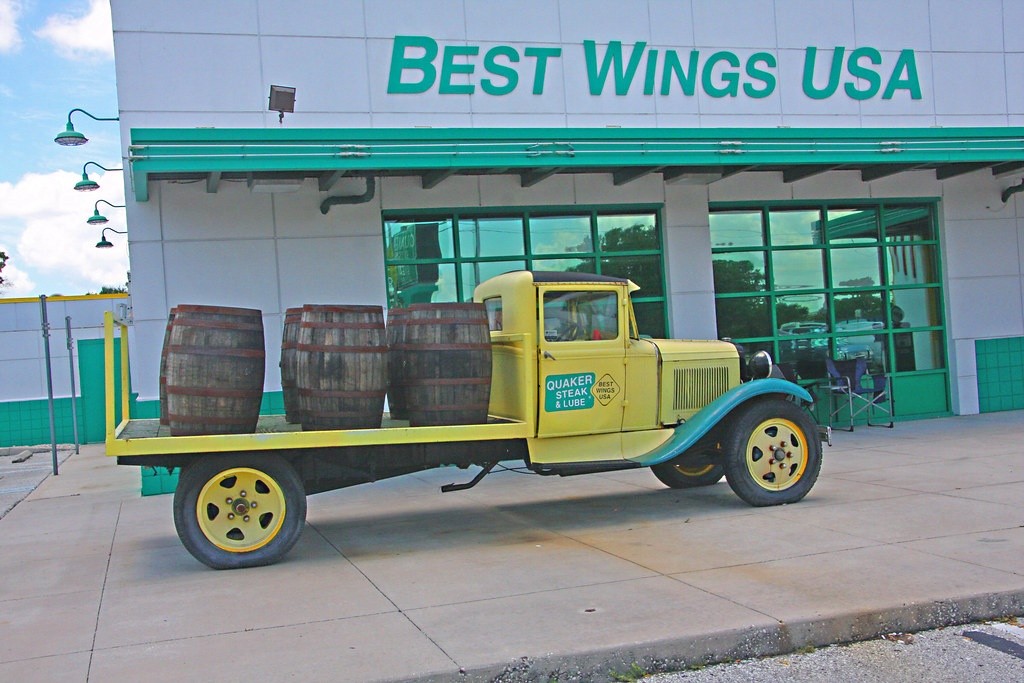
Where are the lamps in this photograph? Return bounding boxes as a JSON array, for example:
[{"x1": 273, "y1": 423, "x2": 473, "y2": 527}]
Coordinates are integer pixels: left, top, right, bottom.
[
  {"x1": 74, "y1": 161, "x2": 123, "y2": 192},
  {"x1": 87, "y1": 199, "x2": 126, "y2": 226},
  {"x1": 268, "y1": 85, "x2": 296, "y2": 125},
  {"x1": 95, "y1": 227, "x2": 128, "y2": 248},
  {"x1": 54, "y1": 107, "x2": 119, "y2": 146}
]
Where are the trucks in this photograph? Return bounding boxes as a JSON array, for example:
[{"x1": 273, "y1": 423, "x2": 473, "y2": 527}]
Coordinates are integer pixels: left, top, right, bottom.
[{"x1": 103, "y1": 268, "x2": 833, "y2": 571}]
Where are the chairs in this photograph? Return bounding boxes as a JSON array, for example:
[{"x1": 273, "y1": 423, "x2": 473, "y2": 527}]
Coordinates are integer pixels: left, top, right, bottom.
[
  {"x1": 770, "y1": 363, "x2": 822, "y2": 426},
  {"x1": 817, "y1": 353, "x2": 895, "y2": 431}
]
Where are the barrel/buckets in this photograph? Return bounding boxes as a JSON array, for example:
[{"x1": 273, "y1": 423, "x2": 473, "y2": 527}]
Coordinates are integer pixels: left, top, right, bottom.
[
  {"x1": 385, "y1": 308, "x2": 410, "y2": 420},
  {"x1": 166, "y1": 304, "x2": 266, "y2": 435},
  {"x1": 280, "y1": 308, "x2": 302, "y2": 423},
  {"x1": 407, "y1": 302, "x2": 492, "y2": 427},
  {"x1": 160, "y1": 307, "x2": 177, "y2": 425},
  {"x1": 297, "y1": 303, "x2": 388, "y2": 431}
]
